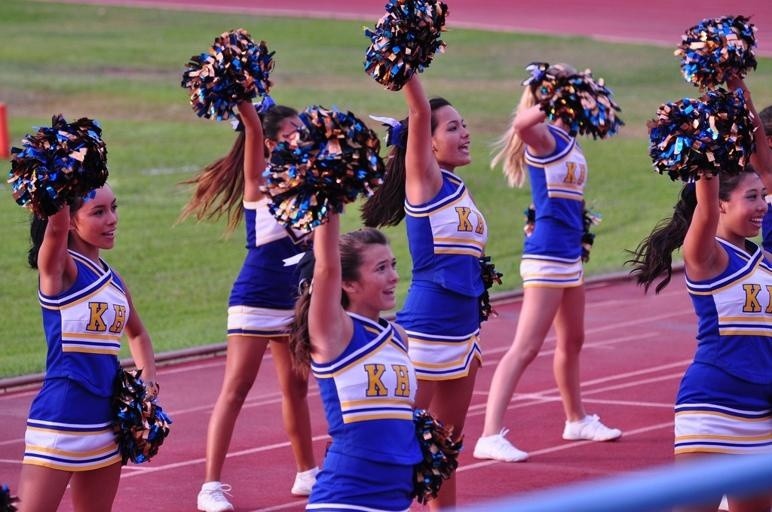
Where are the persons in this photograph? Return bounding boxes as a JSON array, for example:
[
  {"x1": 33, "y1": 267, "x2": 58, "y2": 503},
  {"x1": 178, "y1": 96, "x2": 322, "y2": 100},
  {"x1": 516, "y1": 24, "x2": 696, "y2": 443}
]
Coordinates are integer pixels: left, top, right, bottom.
[
  {"x1": 289, "y1": 215, "x2": 424, "y2": 512},
  {"x1": 170, "y1": 100, "x2": 322, "y2": 512},
  {"x1": 13, "y1": 183, "x2": 158, "y2": 512},
  {"x1": 473, "y1": 63, "x2": 622, "y2": 464},
  {"x1": 726, "y1": 72, "x2": 772, "y2": 194},
  {"x1": 357, "y1": 70, "x2": 488, "y2": 511},
  {"x1": 624, "y1": 158, "x2": 772, "y2": 511}
]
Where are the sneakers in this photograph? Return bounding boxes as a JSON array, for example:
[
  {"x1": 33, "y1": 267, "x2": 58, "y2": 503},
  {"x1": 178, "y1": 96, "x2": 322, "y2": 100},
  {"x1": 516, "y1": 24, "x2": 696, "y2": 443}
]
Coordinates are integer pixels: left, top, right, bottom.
[
  {"x1": 195, "y1": 482, "x2": 235, "y2": 512},
  {"x1": 473, "y1": 426, "x2": 529, "y2": 462},
  {"x1": 290, "y1": 466, "x2": 322, "y2": 496},
  {"x1": 561, "y1": 414, "x2": 622, "y2": 444}
]
